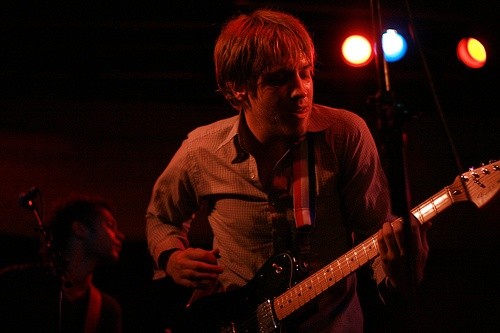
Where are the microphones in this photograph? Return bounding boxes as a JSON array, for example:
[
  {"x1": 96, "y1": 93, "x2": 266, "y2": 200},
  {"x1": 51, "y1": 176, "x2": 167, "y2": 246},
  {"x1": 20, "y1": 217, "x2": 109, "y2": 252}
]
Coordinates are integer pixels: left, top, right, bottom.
[{"x1": 19, "y1": 185, "x2": 39, "y2": 207}]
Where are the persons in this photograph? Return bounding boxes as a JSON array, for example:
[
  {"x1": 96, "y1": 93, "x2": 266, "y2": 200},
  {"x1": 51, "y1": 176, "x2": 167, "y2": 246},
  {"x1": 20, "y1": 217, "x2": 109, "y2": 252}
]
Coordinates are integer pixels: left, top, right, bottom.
[
  {"x1": 0, "y1": 191, "x2": 126, "y2": 333},
  {"x1": 145, "y1": 8, "x2": 434, "y2": 333}
]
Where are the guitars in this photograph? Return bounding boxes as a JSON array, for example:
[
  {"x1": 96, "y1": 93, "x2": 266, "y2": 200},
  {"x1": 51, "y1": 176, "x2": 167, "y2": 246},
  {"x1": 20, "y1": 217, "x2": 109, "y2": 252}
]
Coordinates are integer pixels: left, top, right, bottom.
[{"x1": 162, "y1": 157, "x2": 500, "y2": 333}]
